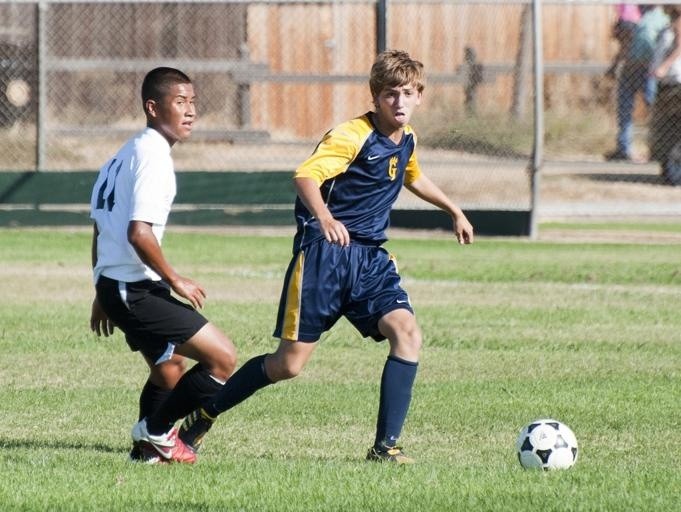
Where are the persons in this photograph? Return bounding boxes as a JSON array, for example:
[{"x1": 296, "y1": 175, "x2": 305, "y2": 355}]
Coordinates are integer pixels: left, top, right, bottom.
[
  {"x1": 178, "y1": 47, "x2": 477, "y2": 466},
  {"x1": 598, "y1": 4, "x2": 680, "y2": 187},
  {"x1": 85, "y1": 66, "x2": 238, "y2": 466}
]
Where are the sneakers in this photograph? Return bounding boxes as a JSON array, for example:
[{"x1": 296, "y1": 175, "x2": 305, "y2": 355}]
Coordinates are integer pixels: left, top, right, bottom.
[
  {"x1": 604, "y1": 151, "x2": 631, "y2": 162},
  {"x1": 178, "y1": 407, "x2": 218, "y2": 453},
  {"x1": 365, "y1": 444, "x2": 419, "y2": 469},
  {"x1": 129, "y1": 417, "x2": 197, "y2": 465}
]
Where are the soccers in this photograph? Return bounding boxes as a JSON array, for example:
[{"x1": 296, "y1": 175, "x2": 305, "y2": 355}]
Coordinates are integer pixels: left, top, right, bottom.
[{"x1": 517, "y1": 419, "x2": 578, "y2": 471}]
